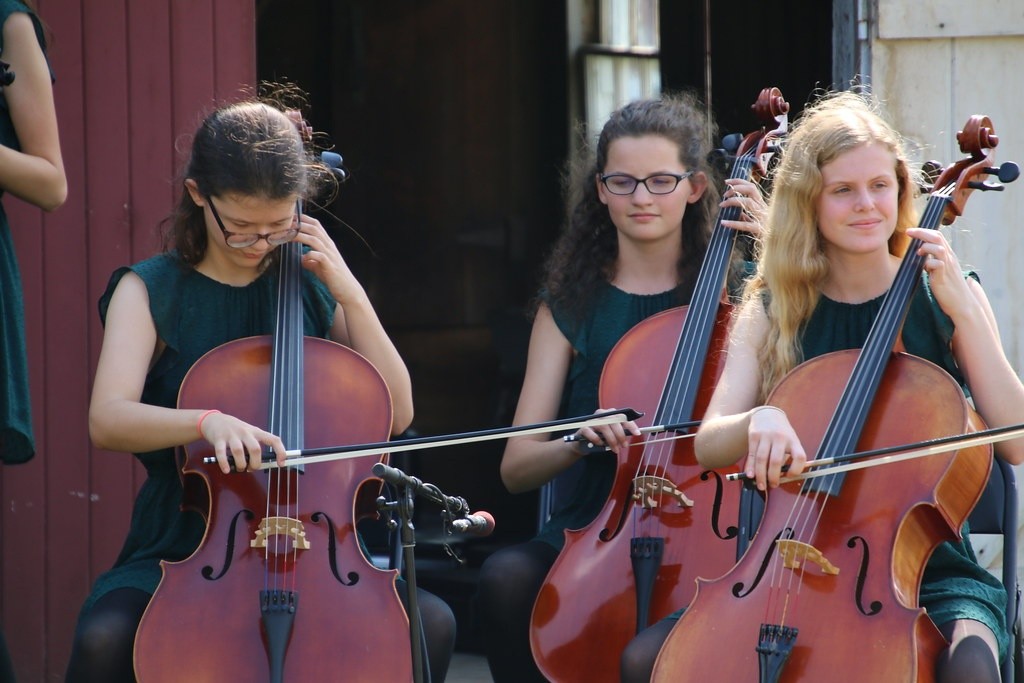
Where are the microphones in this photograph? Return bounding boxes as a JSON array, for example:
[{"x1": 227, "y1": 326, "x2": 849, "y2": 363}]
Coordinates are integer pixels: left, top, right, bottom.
[{"x1": 452, "y1": 510, "x2": 495, "y2": 538}]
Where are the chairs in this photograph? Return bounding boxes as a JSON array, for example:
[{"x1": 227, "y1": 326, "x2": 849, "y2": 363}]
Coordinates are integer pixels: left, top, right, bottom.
[{"x1": 968, "y1": 453, "x2": 1024, "y2": 683}]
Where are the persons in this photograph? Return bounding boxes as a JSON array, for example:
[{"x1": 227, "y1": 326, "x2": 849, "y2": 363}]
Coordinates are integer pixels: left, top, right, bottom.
[
  {"x1": 1, "y1": 0, "x2": 67, "y2": 466},
  {"x1": 71, "y1": 99, "x2": 455, "y2": 683},
  {"x1": 621, "y1": 95, "x2": 1024, "y2": 683},
  {"x1": 480, "y1": 93, "x2": 764, "y2": 683}
]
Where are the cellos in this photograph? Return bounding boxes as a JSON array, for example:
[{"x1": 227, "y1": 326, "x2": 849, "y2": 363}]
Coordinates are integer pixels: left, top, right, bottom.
[
  {"x1": 132, "y1": 103, "x2": 415, "y2": 683},
  {"x1": 529, "y1": 84, "x2": 790, "y2": 683},
  {"x1": 650, "y1": 114, "x2": 1021, "y2": 683}
]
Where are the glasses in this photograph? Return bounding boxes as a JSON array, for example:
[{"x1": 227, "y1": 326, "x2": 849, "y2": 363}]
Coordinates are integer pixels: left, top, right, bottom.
[
  {"x1": 599, "y1": 171, "x2": 693, "y2": 194},
  {"x1": 205, "y1": 193, "x2": 303, "y2": 249}
]
